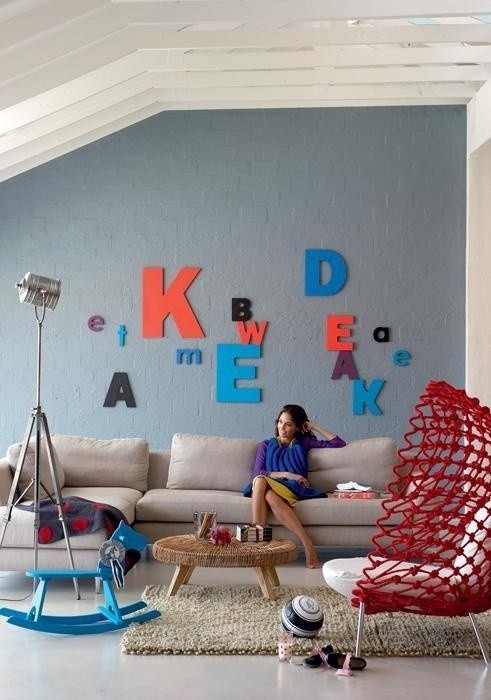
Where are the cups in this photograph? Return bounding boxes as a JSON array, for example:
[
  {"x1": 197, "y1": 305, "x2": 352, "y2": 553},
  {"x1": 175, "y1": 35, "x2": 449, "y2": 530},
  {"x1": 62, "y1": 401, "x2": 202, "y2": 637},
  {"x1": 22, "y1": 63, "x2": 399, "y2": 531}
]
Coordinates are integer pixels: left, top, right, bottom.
[{"x1": 276, "y1": 631, "x2": 293, "y2": 663}]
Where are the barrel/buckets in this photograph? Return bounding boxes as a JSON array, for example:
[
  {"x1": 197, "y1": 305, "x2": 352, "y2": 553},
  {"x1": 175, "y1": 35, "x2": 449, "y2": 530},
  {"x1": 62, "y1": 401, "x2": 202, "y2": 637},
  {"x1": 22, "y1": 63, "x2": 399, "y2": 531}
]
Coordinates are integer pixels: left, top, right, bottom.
[{"x1": 192, "y1": 511, "x2": 217, "y2": 542}]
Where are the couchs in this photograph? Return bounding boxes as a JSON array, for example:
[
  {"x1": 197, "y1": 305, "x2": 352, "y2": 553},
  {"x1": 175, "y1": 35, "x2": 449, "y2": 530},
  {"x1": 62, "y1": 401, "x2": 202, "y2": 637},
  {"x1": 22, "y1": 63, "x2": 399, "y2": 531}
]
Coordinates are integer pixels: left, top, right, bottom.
[{"x1": 2, "y1": 430, "x2": 437, "y2": 595}]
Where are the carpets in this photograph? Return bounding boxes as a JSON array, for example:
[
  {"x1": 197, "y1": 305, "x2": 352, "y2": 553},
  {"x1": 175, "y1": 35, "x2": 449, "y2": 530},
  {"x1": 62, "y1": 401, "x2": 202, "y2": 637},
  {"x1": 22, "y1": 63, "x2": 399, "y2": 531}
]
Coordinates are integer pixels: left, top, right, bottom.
[{"x1": 120, "y1": 583, "x2": 491, "y2": 659}]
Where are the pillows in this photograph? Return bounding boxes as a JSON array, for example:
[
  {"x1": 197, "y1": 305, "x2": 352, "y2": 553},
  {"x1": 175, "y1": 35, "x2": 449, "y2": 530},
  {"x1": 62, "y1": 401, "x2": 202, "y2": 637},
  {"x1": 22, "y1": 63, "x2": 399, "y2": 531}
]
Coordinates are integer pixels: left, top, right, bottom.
[
  {"x1": 304, "y1": 436, "x2": 399, "y2": 497},
  {"x1": 4, "y1": 433, "x2": 67, "y2": 503},
  {"x1": 47, "y1": 433, "x2": 152, "y2": 495},
  {"x1": 165, "y1": 431, "x2": 261, "y2": 494}
]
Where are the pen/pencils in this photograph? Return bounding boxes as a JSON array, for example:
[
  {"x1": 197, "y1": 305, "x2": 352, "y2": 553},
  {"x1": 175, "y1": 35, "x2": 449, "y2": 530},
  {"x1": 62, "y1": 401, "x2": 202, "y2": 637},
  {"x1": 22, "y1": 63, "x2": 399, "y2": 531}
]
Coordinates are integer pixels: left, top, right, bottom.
[{"x1": 195, "y1": 511, "x2": 217, "y2": 540}]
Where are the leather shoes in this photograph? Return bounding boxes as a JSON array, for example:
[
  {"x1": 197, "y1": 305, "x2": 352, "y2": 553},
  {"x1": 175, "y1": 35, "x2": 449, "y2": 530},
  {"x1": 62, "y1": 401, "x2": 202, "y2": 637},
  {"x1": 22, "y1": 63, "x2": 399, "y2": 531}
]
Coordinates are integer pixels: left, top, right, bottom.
[
  {"x1": 324, "y1": 651, "x2": 367, "y2": 671},
  {"x1": 302, "y1": 644, "x2": 334, "y2": 666}
]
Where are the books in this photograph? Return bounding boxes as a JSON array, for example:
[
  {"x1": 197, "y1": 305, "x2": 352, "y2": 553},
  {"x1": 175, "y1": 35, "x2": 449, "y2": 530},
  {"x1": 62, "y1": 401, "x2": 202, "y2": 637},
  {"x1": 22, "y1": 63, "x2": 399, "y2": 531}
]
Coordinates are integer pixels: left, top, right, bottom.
[{"x1": 332, "y1": 480, "x2": 378, "y2": 499}]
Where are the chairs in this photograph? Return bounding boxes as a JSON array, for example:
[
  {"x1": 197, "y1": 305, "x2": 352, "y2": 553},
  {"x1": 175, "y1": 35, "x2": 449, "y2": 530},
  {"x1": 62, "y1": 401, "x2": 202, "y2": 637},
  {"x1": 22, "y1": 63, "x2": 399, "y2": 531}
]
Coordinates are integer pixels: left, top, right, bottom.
[{"x1": 321, "y1": 380, "x2": 491, "y2": 668}]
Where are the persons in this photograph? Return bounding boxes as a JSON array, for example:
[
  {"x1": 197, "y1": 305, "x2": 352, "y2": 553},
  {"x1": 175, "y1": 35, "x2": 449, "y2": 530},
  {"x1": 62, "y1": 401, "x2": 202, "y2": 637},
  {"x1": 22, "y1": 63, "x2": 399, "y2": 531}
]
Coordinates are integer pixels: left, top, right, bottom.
[{"x1": 249, "y1": 404, "x2": 348, "y2": 570}]
